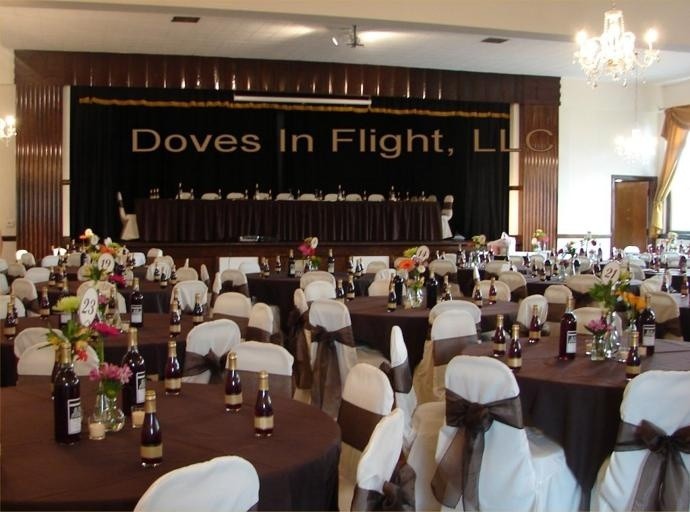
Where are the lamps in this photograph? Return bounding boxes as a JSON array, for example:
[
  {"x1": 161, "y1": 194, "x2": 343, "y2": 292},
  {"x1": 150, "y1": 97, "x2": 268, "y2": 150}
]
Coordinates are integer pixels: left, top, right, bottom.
[
  {"x1": 570, "y1": 1, "x2": 665, "y2": 92},
  {"x1": 0, "y1": 113, "x2": 17, "y2": 140}
]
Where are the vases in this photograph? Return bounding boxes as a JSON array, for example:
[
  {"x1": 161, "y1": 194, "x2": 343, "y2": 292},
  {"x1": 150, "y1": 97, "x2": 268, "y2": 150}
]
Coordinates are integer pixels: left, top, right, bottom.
[{"x1": 100, "y1": 400, "x2": 125, "y2": 432}]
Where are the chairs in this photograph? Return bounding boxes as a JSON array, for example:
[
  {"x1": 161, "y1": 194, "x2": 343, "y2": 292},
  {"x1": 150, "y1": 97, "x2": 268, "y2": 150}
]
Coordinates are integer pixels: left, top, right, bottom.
[
  {"x1": 177, "y1": 189, "x2": 389, "y2": 203},
  {"x1": 389, "y1": 325, "x2": 448, "y2": 512},
  {"x1": 588, "y1": 369, "x2": 690, "y2": 512},
  {"x1": 113, "y1": 187, "x2": 141, "y2": 241},
  {"x1": 1, "y1": 229, "x2": 689, "y2": 414},
  {"x1": 350, "y1": 406, "x2": 415, "y2": 512},
  {"x1": 442, "y1": 195, "x2": 460, "y2": 238},
  {"x1": 132, "y1": 455, "x2": 260, "y2": 512},
  {"x1": 335, "y1": 363, "x2": 396, "y2": 512},
  {"x1": 431, "y1": 356, "x2": 588, "y2": 512}
]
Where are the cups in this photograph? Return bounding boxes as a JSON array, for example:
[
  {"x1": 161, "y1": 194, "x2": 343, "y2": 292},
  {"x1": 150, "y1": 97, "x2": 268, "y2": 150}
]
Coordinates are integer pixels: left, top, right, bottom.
[
  {"x1": 585, "y1": 339, "x2": 594, "y2": 355},
  {"x1": 87, "y1": 415, "x2": 106, "y2": 440},
  {"x1": 130, "y1": 404, "x2": 144, "y2": 428}
]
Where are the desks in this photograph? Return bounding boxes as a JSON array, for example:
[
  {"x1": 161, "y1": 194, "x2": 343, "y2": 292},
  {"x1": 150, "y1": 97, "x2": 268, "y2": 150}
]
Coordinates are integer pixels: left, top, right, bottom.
[{"x1": 135, "y1": 197, "x2": 440, "y2": 243}]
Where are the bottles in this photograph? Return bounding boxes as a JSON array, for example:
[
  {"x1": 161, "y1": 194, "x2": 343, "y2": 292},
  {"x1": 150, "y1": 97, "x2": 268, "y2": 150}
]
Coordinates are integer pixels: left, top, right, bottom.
[
  {"x1": 121, "y1": 328, "x2": 145, "y2": 417},
  {"x1": 163, "y1": 341, "x2": 182, "y2": 395},
  {"x1": 55, "y1": 342, "x2": 82, "y2": 444},
  {"x1": 627, "y1": 332, "x2": 641, "y2": 382},
  {"x1": 505, "y1": 324, "x2": 523, "y2": 372},
  {"x1": 49, "y1": 350, "x2": 61, "y2": 399},
  {"x1": 148, "y1": 184, "x2": 427, "y2": 202},
  {"x1": 223, "y1": 351, "x2": 243, "y2": 412},
  {"x1": 559, "y1": 295, "x2": 577, "y2": 359},
  {"x1": 529, "y1": 305, "x2": 541, "y2": 344},
  {"x1": 139, "y1": 389, "x2": 162, "y2": 469},
  {"x1": 493, "y1": 314, "x2": 506, "y2": 358},
  {"x1": 639, "y1": 293, "x2": 656, "y2": 355},
  {"x1": 3, "y1": 241, "x2": 204, "y2": 342},
  {"x1": 254, "y1": 369, "x2": 274, "y2": 436},
  {"x1": 509, "y1": 241, "x2": 689, "y2": 299},
  {"x1": 258, "y1": 244, "x2": 498, "y2": 310}
]
